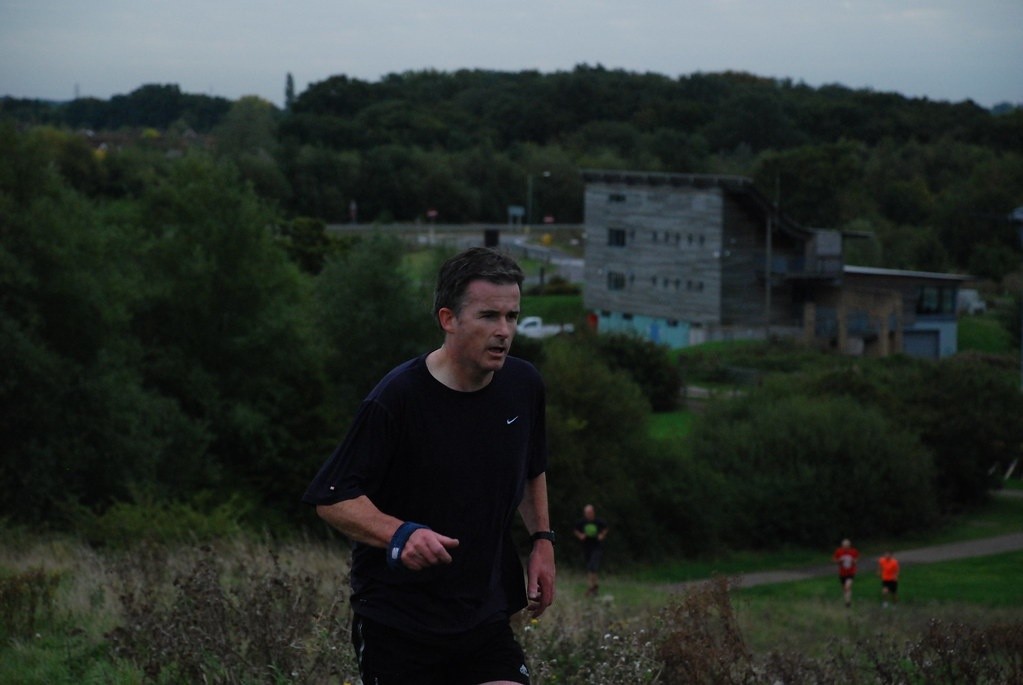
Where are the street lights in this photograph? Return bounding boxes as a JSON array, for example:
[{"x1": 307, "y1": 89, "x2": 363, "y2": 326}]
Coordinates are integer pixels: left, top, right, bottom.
[{"x1": 525, "y1": 171, "x2": 551, "y2": 237}]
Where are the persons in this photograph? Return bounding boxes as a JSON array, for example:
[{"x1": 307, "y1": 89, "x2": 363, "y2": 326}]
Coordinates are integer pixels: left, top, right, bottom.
[
  {"x1": 877, "y1": 550, "x2": 900, "y2": 600},
  {"x1": 574, "y1": 504, "x2": 608, "y2": 596},
  {"x1": 303, "y1": 248, "x2": 557, "y2": 685},
  {"x1": 832, "y1": 539, "x2": 859, "y2": 605}
]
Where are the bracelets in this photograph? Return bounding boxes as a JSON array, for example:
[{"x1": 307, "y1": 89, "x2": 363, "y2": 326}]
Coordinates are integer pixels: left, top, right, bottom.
[
  {"x1": 388, "y1": 521, "x2": 427, "y2": 565},
  {"x1": 532, "y1": 530, "x2": 556, "y2": 544}
]
